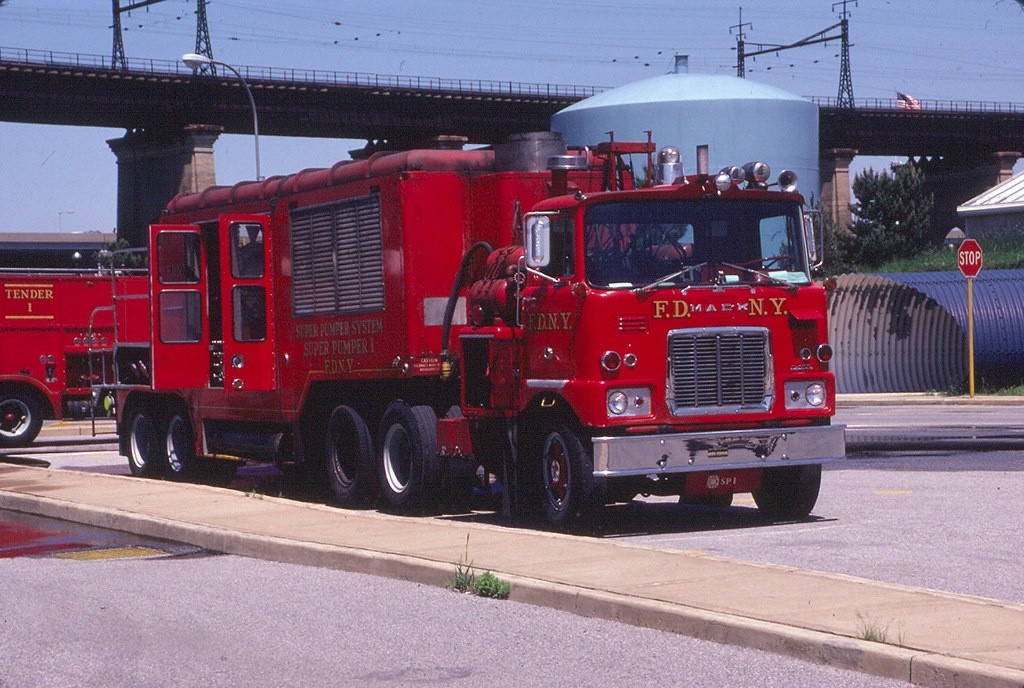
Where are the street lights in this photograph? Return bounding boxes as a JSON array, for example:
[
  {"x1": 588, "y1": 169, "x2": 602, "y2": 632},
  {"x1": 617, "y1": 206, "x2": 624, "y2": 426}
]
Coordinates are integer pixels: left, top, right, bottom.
[{"x1": 182, "y1": 53, "x2": 264, "y2": 184}]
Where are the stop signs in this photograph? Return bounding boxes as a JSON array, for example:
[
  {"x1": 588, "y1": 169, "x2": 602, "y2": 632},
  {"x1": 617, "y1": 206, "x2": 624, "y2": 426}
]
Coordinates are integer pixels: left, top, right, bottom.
[{"x1": 956, "y1": 239, "x2": 983, "y2": 278}]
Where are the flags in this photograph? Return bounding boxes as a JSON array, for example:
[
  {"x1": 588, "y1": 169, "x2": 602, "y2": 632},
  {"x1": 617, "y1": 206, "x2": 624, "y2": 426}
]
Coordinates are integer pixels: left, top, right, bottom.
[{"x1": 896, "y1": 91, "x2": 921, "y2": 110}]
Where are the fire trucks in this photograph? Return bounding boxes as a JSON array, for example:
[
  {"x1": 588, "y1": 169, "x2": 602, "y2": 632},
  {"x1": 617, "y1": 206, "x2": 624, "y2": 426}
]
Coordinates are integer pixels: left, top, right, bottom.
[
  {"x1": 0, "y1": 267, "x2": 189, "y2": 446},
  {"x1": 87, "y1": 131, "x2": 848, "y2": 532}
]
